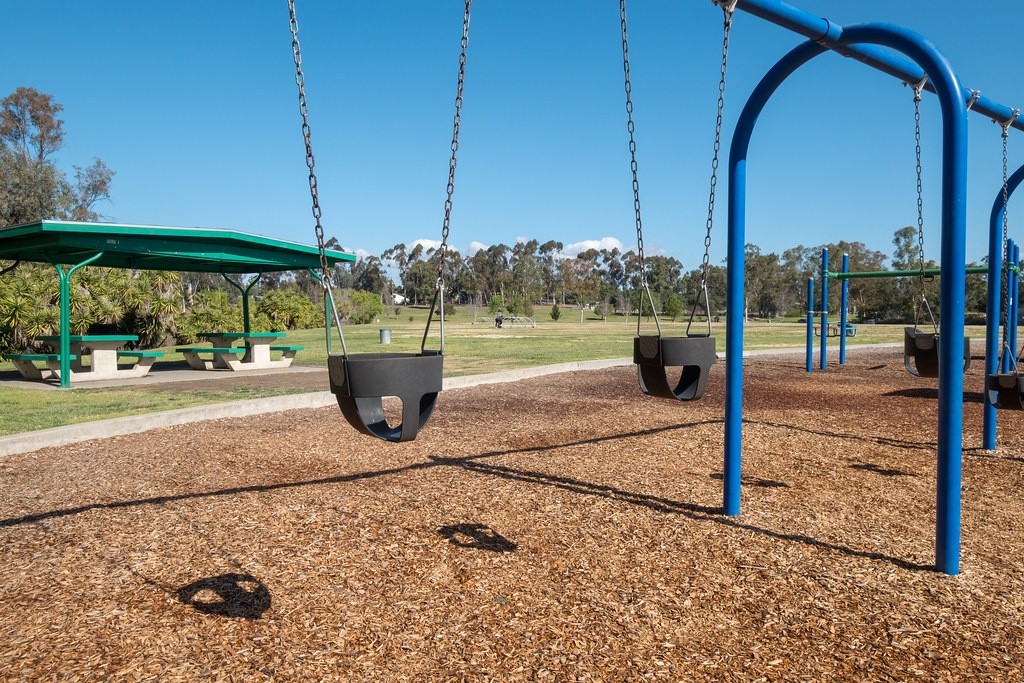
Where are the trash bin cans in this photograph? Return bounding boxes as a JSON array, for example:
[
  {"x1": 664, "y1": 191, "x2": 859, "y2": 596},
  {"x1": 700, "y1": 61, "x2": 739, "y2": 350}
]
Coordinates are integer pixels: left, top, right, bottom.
[{"x1": 380, "y1": 328, "x2": 391, "y2": 345}]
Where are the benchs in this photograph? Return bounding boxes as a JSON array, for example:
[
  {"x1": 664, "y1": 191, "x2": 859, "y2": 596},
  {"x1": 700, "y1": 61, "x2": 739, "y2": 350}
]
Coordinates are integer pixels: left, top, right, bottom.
[
  {"x1": 5, "y1": 353, "x2": 80, "y2": 382},
  {"x1": 813, "y1": 325, "x2": 835, "y2": 336},
  {"x1": 174, "y1": 346, "x2": 246, "y2": 372},
  {"x1": 236, "y1": 345, "x2": 304, "y2": 368},
  {"x1": 117, "y1": 350, "x2": 164, "y2": 378},
  {"x1": 836, "y1": 327, "x2": 858, "y2": 336}
]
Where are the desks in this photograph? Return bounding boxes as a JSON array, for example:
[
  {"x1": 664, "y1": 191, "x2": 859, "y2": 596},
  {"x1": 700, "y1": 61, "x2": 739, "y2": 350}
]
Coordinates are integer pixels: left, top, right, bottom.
[
  {"x1": 195, "y1": 331, "x2": 286, "y2": 371},
  {"x1": 819, "y1": 321, "x2": 850, "y2": 336},
  {"x1": 33, "y1": 333, "x2": 138, "y2": 381}
]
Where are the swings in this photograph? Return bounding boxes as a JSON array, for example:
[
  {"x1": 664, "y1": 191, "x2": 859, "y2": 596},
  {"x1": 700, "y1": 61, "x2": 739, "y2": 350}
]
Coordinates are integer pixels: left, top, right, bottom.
[
  {"x1": 986, "y1": 137, "x2": 1024, "y2": 410},
  {"x1": 287, "y1": 0, "x2": 472, "y2": 443},
  {"x1": 619, "y1": 0, "x2": 737, "y2": 402},
  {"x1": 904, "y1": 101, "x2": 970, "y2": 378}
]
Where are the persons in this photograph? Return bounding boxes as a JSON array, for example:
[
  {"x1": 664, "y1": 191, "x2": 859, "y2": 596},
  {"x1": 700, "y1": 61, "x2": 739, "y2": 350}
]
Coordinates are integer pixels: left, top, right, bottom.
[{"x1": 496, "y1": 310, "x2": 502, "y2": 328}]
